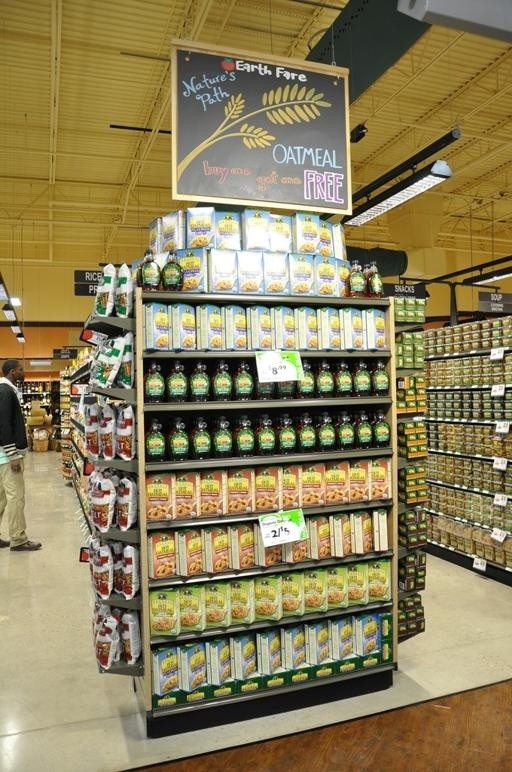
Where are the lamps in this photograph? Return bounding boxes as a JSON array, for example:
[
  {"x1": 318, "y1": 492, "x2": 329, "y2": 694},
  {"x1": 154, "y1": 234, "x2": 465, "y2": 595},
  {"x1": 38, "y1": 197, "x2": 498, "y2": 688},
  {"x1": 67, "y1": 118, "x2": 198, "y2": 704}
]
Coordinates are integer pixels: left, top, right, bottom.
[
  {"x1": 0, "y1": 271, "x2": 28, "y2": 343},
  {"x1": 340, "y1": 160, "x2": 452, "y2": 227}
]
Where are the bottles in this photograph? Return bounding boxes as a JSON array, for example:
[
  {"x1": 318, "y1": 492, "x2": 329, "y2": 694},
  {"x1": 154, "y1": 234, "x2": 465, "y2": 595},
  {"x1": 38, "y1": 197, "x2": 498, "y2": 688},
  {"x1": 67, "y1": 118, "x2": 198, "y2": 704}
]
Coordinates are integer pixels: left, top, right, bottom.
[
  {"x1": 137, "y1": 248, "x2": 161, "y2": 292},
  {"x1": 145, "y1": 360, "x2": 391, "y2": 463},
  {"x1": 346, "y1": 259, "x2": 385, "y2": 299},
  {"x1": 161, "y1": 248, "x2": 185, "y2": 291}
]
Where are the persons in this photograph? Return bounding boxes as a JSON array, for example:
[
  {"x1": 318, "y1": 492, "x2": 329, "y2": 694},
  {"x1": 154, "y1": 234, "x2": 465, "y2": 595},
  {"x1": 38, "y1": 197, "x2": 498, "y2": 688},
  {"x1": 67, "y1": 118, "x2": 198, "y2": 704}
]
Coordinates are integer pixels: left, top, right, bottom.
[{"x1": 0, "y1": 359, "x2": 43, "y2": 552}]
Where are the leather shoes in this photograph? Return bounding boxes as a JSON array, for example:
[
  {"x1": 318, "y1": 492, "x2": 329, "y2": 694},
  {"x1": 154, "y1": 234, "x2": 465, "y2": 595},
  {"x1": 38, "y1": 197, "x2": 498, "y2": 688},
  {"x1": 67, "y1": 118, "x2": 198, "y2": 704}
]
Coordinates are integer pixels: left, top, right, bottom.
[
  {"x1": 10, "y1": 540, "x2": 42, "y2": 551},
  {"x1": 0, "y1": 539, "x2": 10, "y2": 548}
]
